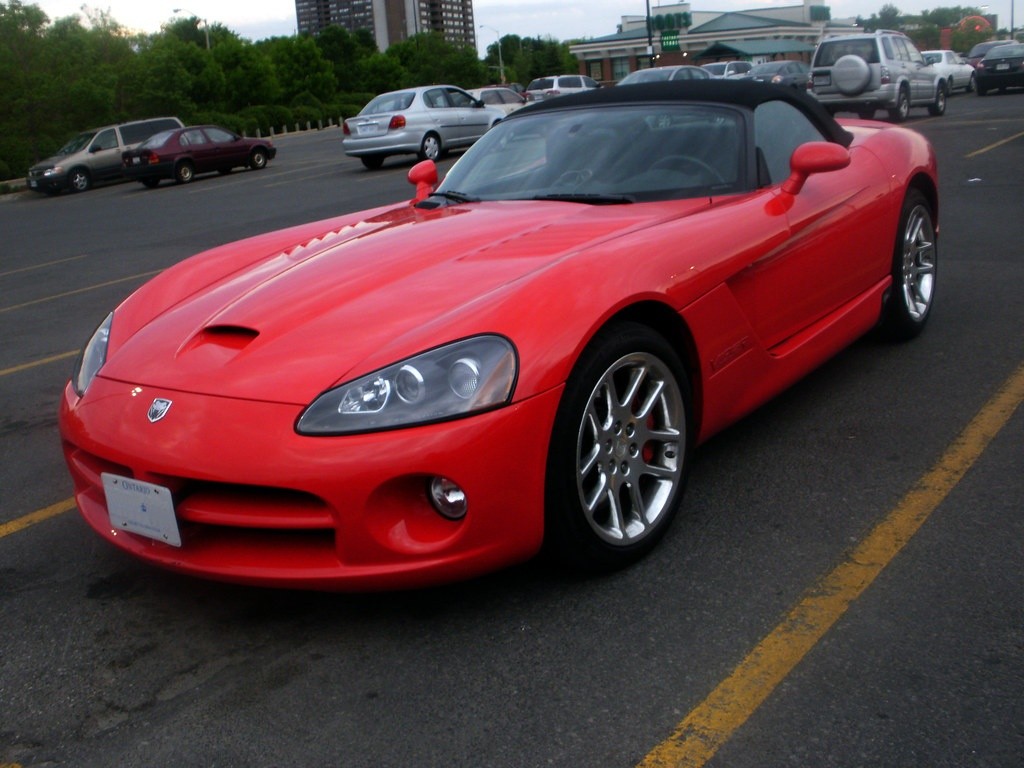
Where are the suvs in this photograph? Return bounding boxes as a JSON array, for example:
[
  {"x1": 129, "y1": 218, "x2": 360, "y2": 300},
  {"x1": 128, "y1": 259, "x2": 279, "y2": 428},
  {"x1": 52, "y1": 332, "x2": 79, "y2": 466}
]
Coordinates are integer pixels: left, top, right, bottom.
[{"x1": 806, "y1": 28, "x2": 949, "y2": 120}]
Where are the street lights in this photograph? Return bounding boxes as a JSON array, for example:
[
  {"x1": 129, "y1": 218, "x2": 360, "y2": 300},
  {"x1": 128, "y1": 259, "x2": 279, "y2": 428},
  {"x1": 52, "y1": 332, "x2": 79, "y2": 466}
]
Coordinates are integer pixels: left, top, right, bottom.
[
  {"x1": 174, "y1": 8, "x2": 210, "y2": 48},
  {"x1": 480, "y1": 24, "x2": 505, "y2": 85}
]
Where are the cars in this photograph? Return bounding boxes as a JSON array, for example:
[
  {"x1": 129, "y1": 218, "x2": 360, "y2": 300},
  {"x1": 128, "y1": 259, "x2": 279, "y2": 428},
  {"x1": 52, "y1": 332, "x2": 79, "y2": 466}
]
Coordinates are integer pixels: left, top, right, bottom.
[
  {"x1": 978, "y1": 43, "x2": 1024, "y2": 94},
  {"x1": 526, "y1": 74, "x2": 604, "y2": 101},
  {"x1": 701, "y1": 61, "x2": 753, "y2": 82},
  {"x1": 920, "y1": 50, "x2": 977, "y2": 92},
  {"x1": 966, "y1": 39, "x2": 1020, "y2": 66},
  {"x1": 486, "y1": 83, "x2": 527, "y2": 101},
  {"x1": 122, "y1": 124, "x2": 277, "y2": 188},
  {"x1": 26, "y1": 116, "x2": 187, "y2": 197},
  {"x1": 614, "y1": 65, "x2": 728, "y2": 127},
  {"x1": 340, "y1": 84, "x2": 504, "y2": 169},
  {"x1": 437, "y1": 88, "x2": 542, "y2": 116},
  {"x1": 739, "y1": 60, "x2": 810, "y2": 90}
]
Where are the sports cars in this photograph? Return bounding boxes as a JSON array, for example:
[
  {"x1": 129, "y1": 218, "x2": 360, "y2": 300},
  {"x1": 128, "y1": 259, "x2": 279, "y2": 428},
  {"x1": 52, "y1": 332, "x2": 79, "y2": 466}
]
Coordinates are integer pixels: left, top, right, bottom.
[{"x1": 59, "y1": 78, "x2": 942, "y2": 588}]
